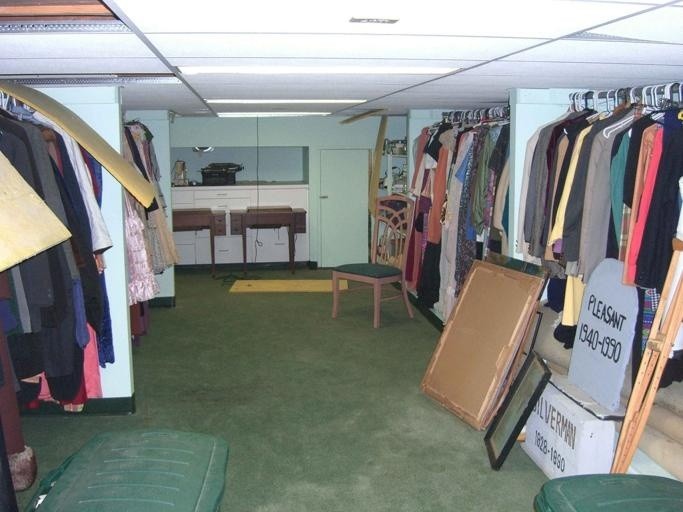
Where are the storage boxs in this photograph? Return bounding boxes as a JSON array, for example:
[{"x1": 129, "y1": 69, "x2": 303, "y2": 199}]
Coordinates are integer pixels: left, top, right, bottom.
[{"x1": 525, "y1": 374, "x2": 626, "y2": 476}]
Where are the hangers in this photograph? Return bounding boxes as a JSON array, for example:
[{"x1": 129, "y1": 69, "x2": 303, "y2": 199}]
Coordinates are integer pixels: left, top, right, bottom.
[
  {"x1": 422, "y1": 104, "x2": 511, "y2": 130},
  {"x1": 544, "y1": 84, "x2": 683, "y2": 139}
]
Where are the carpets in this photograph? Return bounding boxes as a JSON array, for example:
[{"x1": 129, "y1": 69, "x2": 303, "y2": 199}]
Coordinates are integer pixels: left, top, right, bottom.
[{"x1": 228, "y1": 278, "x2": 348, "y2": 293}]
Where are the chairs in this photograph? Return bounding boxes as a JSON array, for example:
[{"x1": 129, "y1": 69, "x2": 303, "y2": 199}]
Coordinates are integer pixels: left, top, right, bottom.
[{"x1": 331, "y1": 194, "x2": 414, "y2": 328}]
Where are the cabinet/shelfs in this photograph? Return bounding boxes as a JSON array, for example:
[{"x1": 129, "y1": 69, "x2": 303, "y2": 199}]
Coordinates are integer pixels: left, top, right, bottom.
[
  {"x1": 170, "y1": 184, "x2": 309, "y2": 265},
  {"x1": 378, "y1": 154, "x2": 407, "y2": 240}
]
